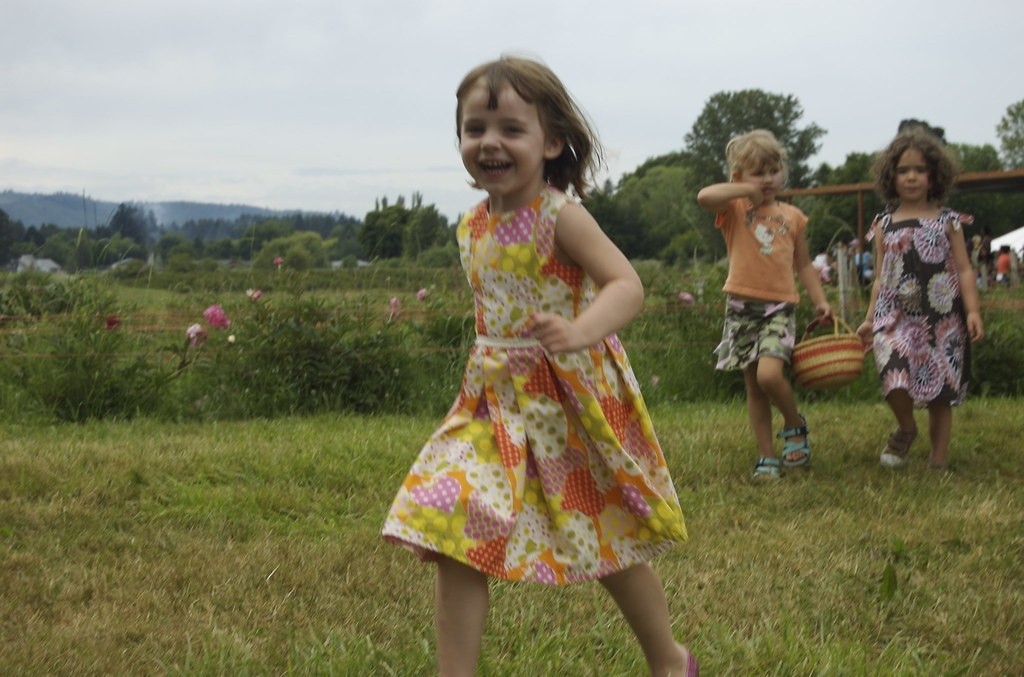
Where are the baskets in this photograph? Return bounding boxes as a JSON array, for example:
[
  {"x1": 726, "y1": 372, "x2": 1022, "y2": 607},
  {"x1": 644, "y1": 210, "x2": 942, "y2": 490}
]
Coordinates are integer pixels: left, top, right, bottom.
[{"x1": 792, "y1": 314, "x2": 865, "y2": 390}]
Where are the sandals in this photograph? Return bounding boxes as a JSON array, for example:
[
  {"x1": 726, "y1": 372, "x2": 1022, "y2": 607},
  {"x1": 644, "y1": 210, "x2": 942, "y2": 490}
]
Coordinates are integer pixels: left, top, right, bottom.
[
  {"x1": 777, "y1": 414, "x2": 811, "y2": 468},
  {"x1": 754, "y1": 457, "x2": 780, "y2": 480}
]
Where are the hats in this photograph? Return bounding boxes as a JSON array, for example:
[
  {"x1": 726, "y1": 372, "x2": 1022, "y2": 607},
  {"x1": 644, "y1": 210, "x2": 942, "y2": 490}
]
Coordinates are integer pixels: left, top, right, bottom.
[{"x1": 849, "y1": 238, "x2": 860, "y2": 245}]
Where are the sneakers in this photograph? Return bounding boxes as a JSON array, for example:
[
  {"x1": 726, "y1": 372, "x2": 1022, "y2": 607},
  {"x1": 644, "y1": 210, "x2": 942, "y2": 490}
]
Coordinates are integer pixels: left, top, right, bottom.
[
  {"x1": 880, "y1": 423, "x2": 917, "y2": 469},
  {"x1": 928, "y1": 458, "x2": 949, "y2": 471}
]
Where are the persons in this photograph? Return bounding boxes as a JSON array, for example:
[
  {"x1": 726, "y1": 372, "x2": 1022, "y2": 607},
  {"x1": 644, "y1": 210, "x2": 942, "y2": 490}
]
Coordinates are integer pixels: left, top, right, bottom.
[
  {"x1": 855, "y1": 130, "x2": 985, "y2": 470},
  {"x1": 382, "y1": 57, "x2": 699, "y2": 677},
  {"x1": 969, "y1": 236, "x2": 1010, "y2": 285},
  {"x1": 698, "y1": 129, "x2": 833, "y2": 480},
  {"x1": 812, "y1": 238, "x2": 873, "y2": 283}
]
